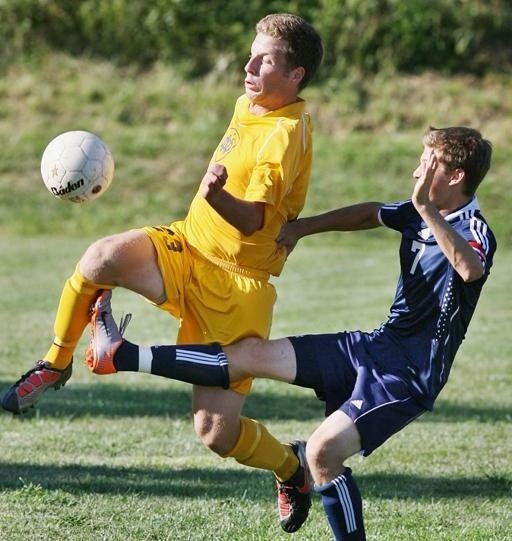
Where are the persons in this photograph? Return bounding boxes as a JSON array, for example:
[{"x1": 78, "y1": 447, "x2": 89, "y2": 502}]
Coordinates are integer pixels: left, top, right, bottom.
[
  {"x1": 83, "y1": 127, "x2": 496, "y2": 541},
  {"x1": 0, "y1": 12, "x2": 323, "y2": 534}
]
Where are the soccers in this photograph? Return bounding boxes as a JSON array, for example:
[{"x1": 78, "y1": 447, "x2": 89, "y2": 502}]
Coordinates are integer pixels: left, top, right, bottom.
[{"x1": 41, "y1": 130, "x2": 116, "y2": 204}]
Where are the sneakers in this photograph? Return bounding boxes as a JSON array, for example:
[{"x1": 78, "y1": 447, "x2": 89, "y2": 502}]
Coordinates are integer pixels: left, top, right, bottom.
[
  {"x1": 275, "y1": 439, "x2": 313, "y2": 533},
  {"x1": 86, "y1": 287, "x2": 125, "y2": 377},
  {"x1": 4, "y1": 357, "x2": 74, "y2": 416}
]
